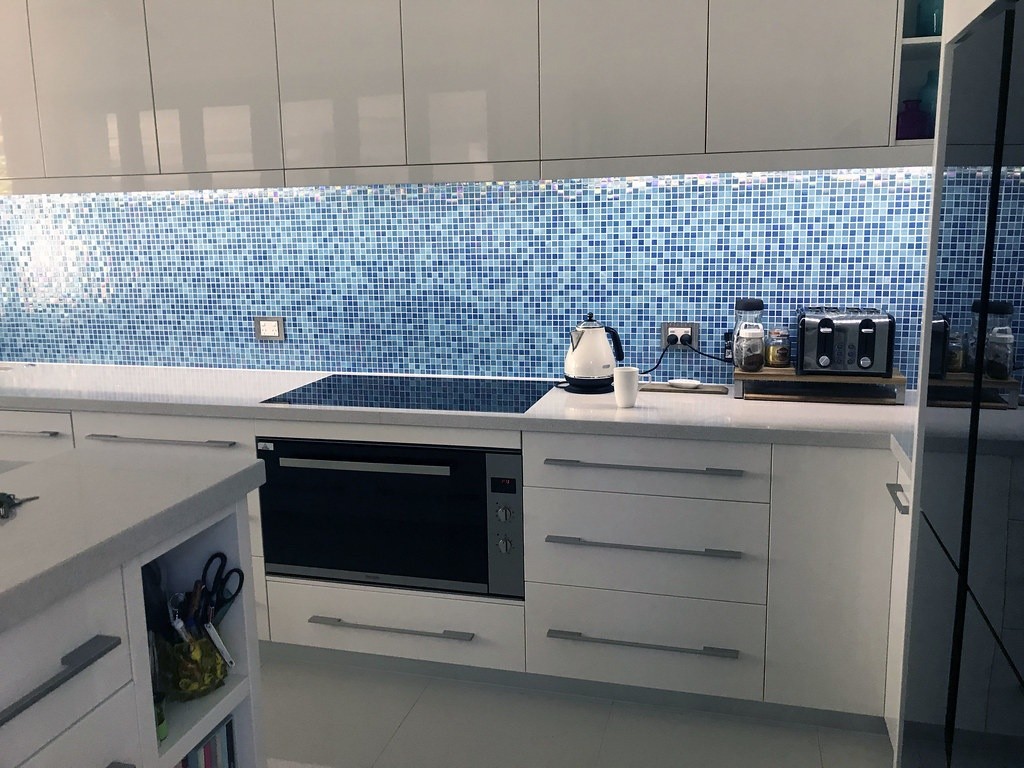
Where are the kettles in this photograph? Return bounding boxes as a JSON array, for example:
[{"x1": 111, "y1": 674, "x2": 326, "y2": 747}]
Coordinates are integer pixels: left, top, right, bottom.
[{"x1": 564, "y1": 313, "x2": 624, "y2": 394}]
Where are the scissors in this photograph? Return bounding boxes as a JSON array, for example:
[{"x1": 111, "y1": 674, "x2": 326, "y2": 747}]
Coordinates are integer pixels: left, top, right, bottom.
[{"x1": 198, "y1": 551, "x2": 245, "y2": 629}]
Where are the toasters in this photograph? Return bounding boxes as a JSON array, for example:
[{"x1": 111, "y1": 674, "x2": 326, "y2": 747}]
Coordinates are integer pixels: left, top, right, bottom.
[{"x1": 795, "y1": 307, "x2": 895, "y2": 376}]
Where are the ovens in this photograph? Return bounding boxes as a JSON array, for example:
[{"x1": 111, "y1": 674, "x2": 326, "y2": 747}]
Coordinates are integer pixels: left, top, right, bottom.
[{"x1": 255, "y1": 437, "x2": 525, "y2": 598}]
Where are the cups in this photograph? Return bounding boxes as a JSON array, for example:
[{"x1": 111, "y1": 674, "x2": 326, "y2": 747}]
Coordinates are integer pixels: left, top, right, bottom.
[
  {"x1": 614, "y1": 367, "x2": 651, "y2": 407},
  {"x1": 739, "y1": 321, "x2": 763, "y2": 372}
]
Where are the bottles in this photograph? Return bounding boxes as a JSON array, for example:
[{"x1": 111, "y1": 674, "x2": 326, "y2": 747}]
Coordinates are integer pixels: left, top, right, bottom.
[{"x1": 733, "y1": 298, "x2": 764, "y2": 367}]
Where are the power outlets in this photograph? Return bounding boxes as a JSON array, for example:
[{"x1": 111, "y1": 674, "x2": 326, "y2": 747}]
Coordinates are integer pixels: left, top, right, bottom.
[
  {"x1": 662, "y1": 322, "x2": 698, "y2": 350},
  {"x1": 255, "y1": 316, "x2": 285, "y2": 341}
]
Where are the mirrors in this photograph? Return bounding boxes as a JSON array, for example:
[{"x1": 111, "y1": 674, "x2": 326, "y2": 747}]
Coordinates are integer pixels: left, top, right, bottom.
[{"x1": 901, "y1": 0, "x2": 1024, "y2": 768}]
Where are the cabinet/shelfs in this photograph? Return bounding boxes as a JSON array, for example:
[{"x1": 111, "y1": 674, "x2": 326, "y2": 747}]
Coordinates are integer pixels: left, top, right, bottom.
[
  {"x1": 0, "y1": 0, "x2": 944, "y2": 196},
  {"x1": 734, "y1": 360, "x2": 906, "y2": 405},
  {"x1": 898, "y1": 394, "x2": 1024, "y2": 768},
  {"x1": 0, "y1": 361, "x2": 925, "y2": 734},
  {"x1": 0, "y1": 434, "x2": 267, "y2": 768}
]
